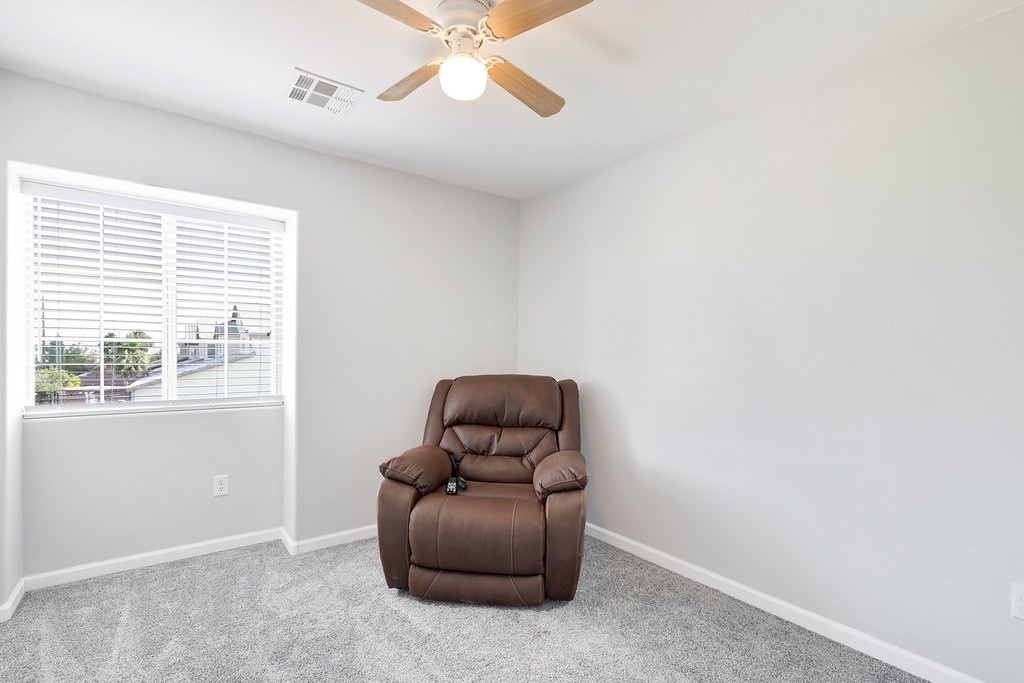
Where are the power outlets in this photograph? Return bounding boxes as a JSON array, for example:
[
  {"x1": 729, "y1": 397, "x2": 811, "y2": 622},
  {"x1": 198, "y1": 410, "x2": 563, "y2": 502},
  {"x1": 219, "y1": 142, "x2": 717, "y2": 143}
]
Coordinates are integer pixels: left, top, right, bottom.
[{"x1": 213, "y1": 475, "x2": 228, "y2": 496}]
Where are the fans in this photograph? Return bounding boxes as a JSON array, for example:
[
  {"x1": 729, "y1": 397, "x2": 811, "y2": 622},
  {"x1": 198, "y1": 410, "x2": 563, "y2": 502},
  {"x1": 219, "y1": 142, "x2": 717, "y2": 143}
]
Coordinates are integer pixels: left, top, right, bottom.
[{"x1": 359, "y1": 0, "x2": 593, "y2": 117}]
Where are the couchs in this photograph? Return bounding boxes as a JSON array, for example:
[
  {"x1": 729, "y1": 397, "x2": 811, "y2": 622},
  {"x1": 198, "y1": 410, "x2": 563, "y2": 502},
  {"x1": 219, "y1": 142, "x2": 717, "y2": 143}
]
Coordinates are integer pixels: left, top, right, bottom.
[{"x1": 377, "y1": 374, "x2": 589, "y2": 606}]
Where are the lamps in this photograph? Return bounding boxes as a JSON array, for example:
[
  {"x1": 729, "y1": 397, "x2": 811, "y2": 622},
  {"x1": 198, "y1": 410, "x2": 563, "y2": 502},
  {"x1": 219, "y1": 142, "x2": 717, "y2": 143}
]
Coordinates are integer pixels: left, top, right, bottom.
[{"x1": 439, "y1": 33, "x2": 487, "y2": 100}]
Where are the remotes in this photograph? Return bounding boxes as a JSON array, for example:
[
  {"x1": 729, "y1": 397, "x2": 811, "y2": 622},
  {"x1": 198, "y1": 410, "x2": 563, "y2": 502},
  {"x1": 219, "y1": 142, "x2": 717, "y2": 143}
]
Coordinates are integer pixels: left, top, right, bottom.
[
  {"x1": 445, "y1": 476, "x2": 457, "y2": 495},
  {"x1": 456, "y1": 476, "x2": 467, "y2": 489}
]
